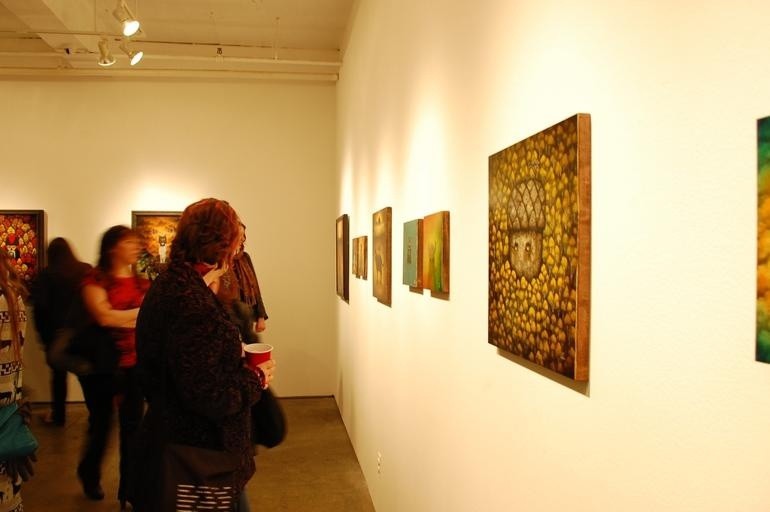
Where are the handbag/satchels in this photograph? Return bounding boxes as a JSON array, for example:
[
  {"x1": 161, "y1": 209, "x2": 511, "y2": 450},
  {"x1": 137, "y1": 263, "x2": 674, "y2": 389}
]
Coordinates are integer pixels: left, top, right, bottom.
[
  {"x1": 161, "y1": 444, "x2": 238, "y2": 511},
  {"x1": 0, "y1": 401, "x2": 38, "y2": 463},
  {"x1": 252, "y1": 389, "x2": 285, "y2": 448}
]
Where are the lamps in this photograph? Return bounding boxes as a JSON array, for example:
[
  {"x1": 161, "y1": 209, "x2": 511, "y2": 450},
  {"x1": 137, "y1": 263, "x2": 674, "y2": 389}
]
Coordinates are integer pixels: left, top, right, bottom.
[{"x1": 96, "y1": 0, "x2": 144, "y2": 69}]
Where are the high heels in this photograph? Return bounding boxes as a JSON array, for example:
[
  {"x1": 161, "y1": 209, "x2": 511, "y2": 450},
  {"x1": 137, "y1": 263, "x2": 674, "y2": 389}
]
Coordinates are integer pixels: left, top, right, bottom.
[
  {"x1": 79, "y1": 472, "x2": 103, "y2": 499},
  {"x1": 117, "y1": 495, "x2": 142, "y2": 511}
]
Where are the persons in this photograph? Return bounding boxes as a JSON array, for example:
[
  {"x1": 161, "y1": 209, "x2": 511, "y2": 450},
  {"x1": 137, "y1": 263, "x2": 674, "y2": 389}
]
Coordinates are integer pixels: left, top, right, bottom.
[
  {"x1": 133, "y1": 196, "x2": 276, "y2": 510},
  {"x1": 76, "y1": 223, "x2": 153, "y2": 501},
  {"x1": 0, "y1": 246, "x2": 30, "y2": 512},
  {"x1": 213, "y1": 217, "x2": 269, "y2": 342},
  {"x1": 29, "y1": 235, "x2": 95, "y2": 428}
]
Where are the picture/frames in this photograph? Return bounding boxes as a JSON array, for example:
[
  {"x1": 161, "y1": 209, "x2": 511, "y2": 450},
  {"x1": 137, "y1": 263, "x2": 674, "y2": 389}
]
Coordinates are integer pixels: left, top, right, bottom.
[
  {"x1": 335, "y1": 214, "x2": 349, "y2": 304},
  {"x1": 0, "y1": 209, "x2": 44, "y2": 285},
  {"x1": 131, "y1": 211, "x2": 183, "y2": 263}
]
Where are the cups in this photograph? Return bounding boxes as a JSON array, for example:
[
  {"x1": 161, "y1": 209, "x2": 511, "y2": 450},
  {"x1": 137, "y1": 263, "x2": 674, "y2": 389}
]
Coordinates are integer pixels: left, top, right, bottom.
[{"x1": 243, "y1": 343, "x2": 274, "y2": 390}]
